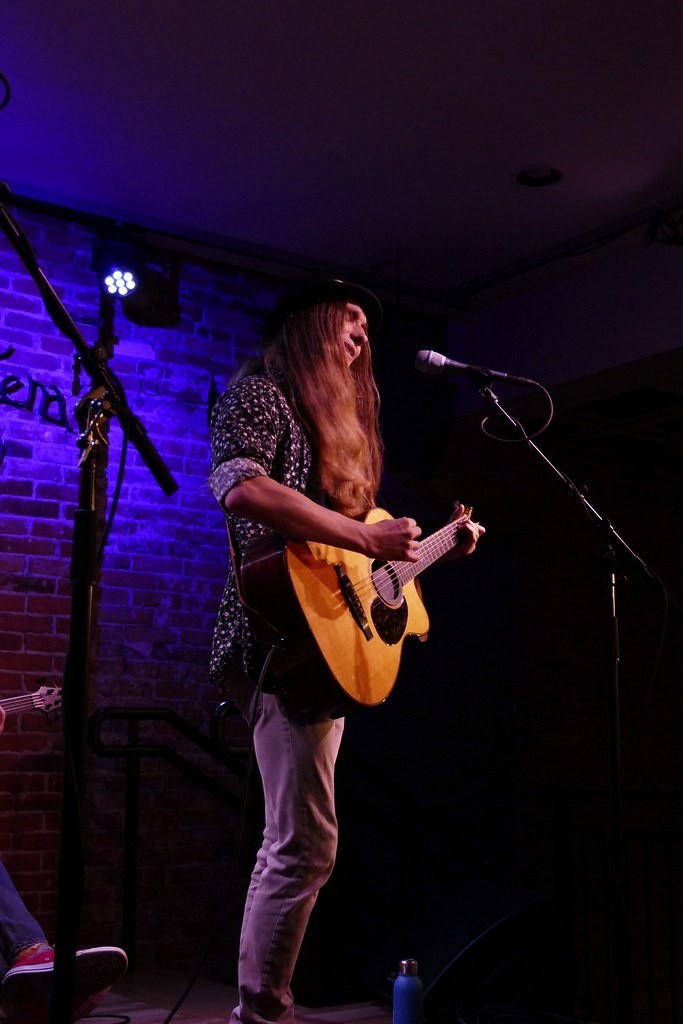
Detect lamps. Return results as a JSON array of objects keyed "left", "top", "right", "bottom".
[{"left": 95, "top": 247, "right": 139, "bottom": 297}]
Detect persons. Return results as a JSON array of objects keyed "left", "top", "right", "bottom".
[
  {"left": 209, "top": 273, "right": 486, "bottom": 1024},
  {"left": 0, "top": 861, "right": 128, "bottom": 1024}
]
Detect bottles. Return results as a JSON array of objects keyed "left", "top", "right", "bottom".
[{"left": 393, "top": 958, "right": 422, "bottom": 1024}]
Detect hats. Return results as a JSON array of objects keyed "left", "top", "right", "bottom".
[{"left": 262, "top": 272, "right": 382, "bottom": 342}]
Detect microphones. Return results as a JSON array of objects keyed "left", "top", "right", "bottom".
[{"left": 414, "top": 348, "right": 539, "bottom": 387}]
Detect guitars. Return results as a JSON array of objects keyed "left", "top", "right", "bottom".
[
  {"left": 0, "top": 676, "right": 63, "bottom": 724},
  {"left": 236, "top": 499, "right": 482, "bottom": 730}
]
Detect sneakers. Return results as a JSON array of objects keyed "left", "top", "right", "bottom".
[
  {"left": 2, "top": 943, "right": 129, "bottom": 1000},
  {"left": 0, "top": 985, "right": 112, "bottom": 1024}
]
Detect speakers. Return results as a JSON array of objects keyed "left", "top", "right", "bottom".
[{"left": 423, "top": 871, "right": 631, "bottom": 1024}]
